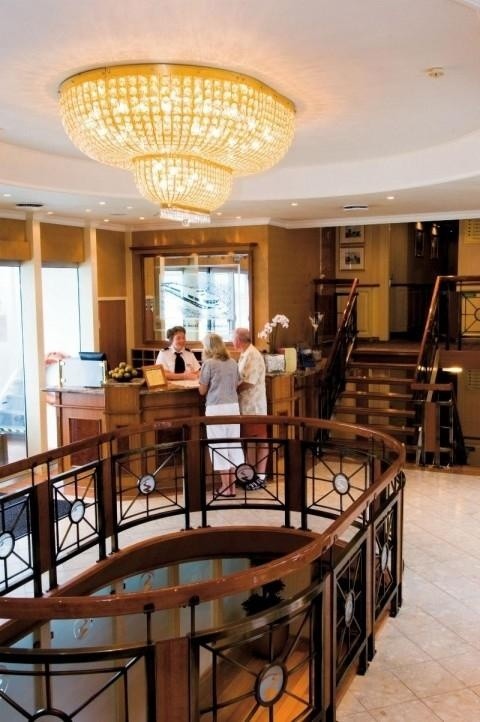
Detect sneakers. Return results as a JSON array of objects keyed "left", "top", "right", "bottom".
[{"left": 245, "top": 475, "right": 267, "bottom": 491}]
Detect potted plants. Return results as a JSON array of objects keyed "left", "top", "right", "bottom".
[{"left": 240, "top": 560, "right": 289, "bottom": 664}]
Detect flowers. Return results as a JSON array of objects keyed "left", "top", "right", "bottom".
[
  {"left": 258, "top": 314, "right": 290, "bottom": 354},
  {"left": 308, "top": 312, "right": 326, "bottom": 351}
]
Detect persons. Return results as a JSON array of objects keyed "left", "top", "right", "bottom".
[
  {"left": 156, "top": 326, "right": 201, "bottom": 381},
  {"left": 230, "top": 328, "right": 270, "bottom": 490},
  {"left": 198, "top": 332, "right": 245, "bottom": 497}
]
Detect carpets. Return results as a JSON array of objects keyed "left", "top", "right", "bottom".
[{"left": 0, "top": 493, "right": 91, "bottom": 541}]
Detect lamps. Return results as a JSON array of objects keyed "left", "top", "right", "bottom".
[{"left": 58, "top": 63, "right": 297, "bottom": 222}]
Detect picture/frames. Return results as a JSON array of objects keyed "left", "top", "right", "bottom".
[
  {"left": 414, "top": 229, "right": 425, "bottom": 257},
  {"left": 338, "top": 225, "right": 366, "bottom": 245},
  {"left": 142, "top": 364, "right": 168, "bottom": 390},
  {"left": 429, "top": 236, "right": 439, "bottom": 260},
  {"left": 339, "top": 245, "right": 365, "bottom": 271}
]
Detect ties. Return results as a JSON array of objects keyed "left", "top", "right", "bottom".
[{"left": 174, "top": 352, "right": 186, "bottom": 374}]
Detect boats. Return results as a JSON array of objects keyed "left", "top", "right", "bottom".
[{"left": 166, "top": 283, "right": 219, "bottom": 310}]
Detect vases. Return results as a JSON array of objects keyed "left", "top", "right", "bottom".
[
  {"left": 311, "top": 349, "right": 323, "bottom": 362},
  {"left": 262, "top": 353, "right": 285, "bottom": 373}
]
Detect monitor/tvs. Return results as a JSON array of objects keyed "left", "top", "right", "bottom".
[{"left": 78, "top": 351, "right": 107, "bottom": 360}]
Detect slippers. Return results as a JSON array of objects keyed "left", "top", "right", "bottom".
[{"left": 213, "top": 488, "right": 237, "bottom": 496}]
